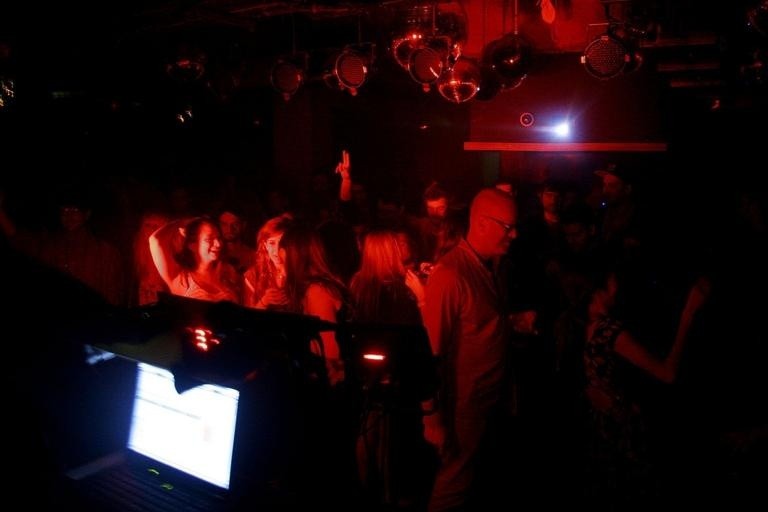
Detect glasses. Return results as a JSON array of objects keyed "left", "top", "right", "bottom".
[{"left": 487, "top": 216, "right": 514, "bottom": 231}]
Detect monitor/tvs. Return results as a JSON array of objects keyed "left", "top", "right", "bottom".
[{"left": 122, "top": 360, "right": 245, "bottom": 500}]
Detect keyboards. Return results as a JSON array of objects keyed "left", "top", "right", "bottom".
[{"left": 88, "top": 461, "right": 219, "bottom": 512}]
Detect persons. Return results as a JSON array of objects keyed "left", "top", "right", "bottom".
[
  {"left": 1, "top": 195, "right": 125, "bottom": 304},
  {"left": 128, "top": 165, "right": 766, "bottom": 512}
]
